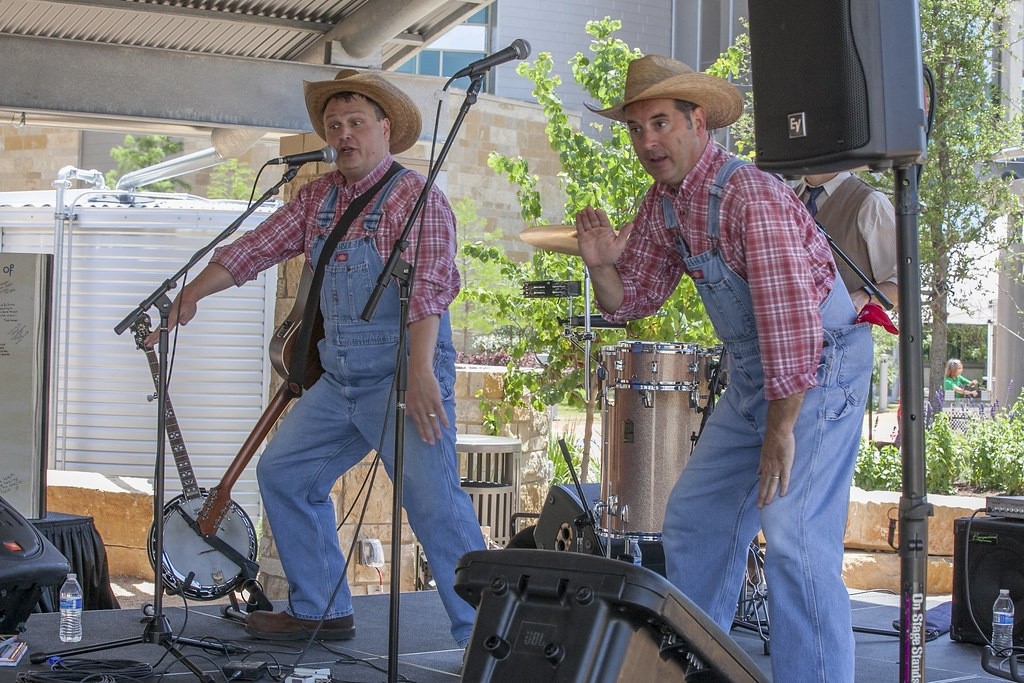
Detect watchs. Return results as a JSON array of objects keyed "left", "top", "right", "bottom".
[{"left": 859, "top": 285, "right": 875, "bottom": 303}]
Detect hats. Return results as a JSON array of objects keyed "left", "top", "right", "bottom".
[
  {"left": 303, "top": 69, "right": 422, "bottom": 156},
  {"left": 582, "top": 55, "right": 743, "bottom": 130}
]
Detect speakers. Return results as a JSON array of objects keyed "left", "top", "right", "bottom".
[
  {"left": 746, "top": 0, "right": 925, "bottom": 179},
  {"left": 949, "top": 515, "right": 1024, "bottom": 651},
  {"left": 453, "top": 548, "right": 770, "bottom": 683},
  {"left": 533, "top": 482, "right": 603, "bottom": 557},
  {"left": 0, "top": 497, "right": 71, "bottom": 635}
]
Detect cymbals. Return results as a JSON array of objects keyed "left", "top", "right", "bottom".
[{"left": 518, "top": 224, "right": 630, "bottom": 256}]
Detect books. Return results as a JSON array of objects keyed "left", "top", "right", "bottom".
[{"left": 0, "top": 635, "right": 28, "bottom": 666}]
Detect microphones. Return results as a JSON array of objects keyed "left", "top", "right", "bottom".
[
  {"left": 454, "top": 39, "right": 531, "bottom": 78},
  {"left": 267, "top": 145, "right": 337, "bottom": 166}
]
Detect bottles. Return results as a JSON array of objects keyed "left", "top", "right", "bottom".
[
  {"left": 627, "top": 539, "right": 642, "bottom": 567},
  {"left": 59, "top": 573, "right": 83, "bottom": 643},
  {"left": 991, "top": 589, "right": 1015, "bottom": 656}
]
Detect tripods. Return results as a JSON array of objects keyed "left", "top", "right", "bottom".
[{"left": 27, "top": 165, "right": 303, "bottom": 683}]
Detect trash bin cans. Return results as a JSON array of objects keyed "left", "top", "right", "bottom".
[{"left": 453, "top": 433, "right": 521, "bottom": 552}]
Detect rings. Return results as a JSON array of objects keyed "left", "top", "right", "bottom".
[
  {"left": 427, "top": 413, "right": 437, "bottom": 417},
  {"left": 771, "top": 476, "right": 781, "bottom": 478}
]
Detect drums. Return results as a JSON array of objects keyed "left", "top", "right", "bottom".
[
  {"left": 593, "top": 345, "right": 725, "bottom": 541},
  {"left": 617, "top": 340, "right": 698, "bottom": 392}
]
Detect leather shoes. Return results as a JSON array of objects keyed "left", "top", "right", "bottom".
[{"left": 244, "top": 610, "right": 356, "bottom": 641}]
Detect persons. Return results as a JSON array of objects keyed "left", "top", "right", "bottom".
[
  {"left": 576, "top": 54, "right": 875, "bottom": 683},
  {"left": 145, "top": 69, "right": 487, "bottom": 649},
  {"left": 743, "top": 170, "right": 898, "bottom": 596},
  {"left": 942, "top": 358, "right": 978, "bottom": 398}
]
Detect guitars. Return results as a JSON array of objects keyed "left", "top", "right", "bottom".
[
  {"left": 129, "top": 313, "right": 258, "bottom": 601},
  {"left": 193, "top": 257, "right": 325, "bottom": 538}
]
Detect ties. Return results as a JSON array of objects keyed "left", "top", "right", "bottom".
[{"left": 805, "top": 185, "right": 825, "bottom": 217}]
